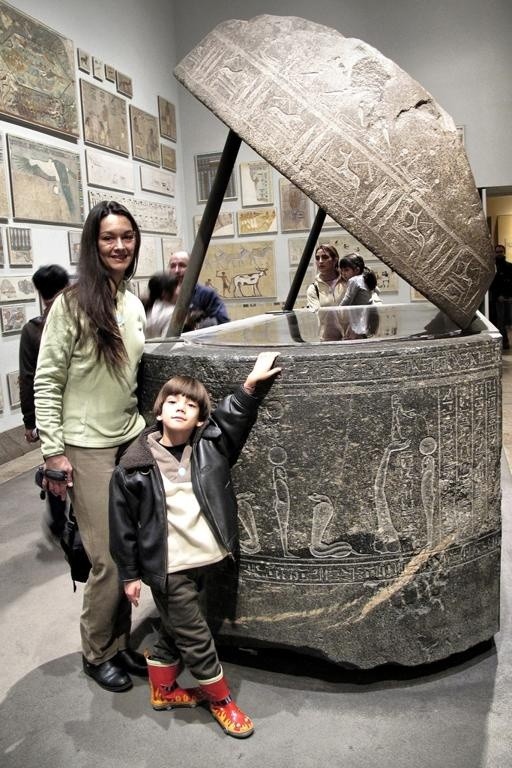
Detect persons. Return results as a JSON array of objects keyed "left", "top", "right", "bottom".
[
  {"left": 132, "top": 248, "right": 230, "bottom": 339},
  {"left": 339, "top": 253, "right": 377, "bottom": 340},
  {"left": 305, "top": 243, "right": 382, "bottom": 342},
  {"left": 34, "top": 200, "right": 148, "bottom": 695},
  {"left": 108, "top": 352, "right": 282, "bottom": 739},
  {"left": 489, "top": 245, "right": 512, "bottom": 350},
  {"left": 19, "top": 264, "right": 70, "bottom": 444}
]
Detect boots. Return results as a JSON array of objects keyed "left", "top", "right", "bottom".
[
  {"left": 145, "top": 655, "right": 206, "bottom": 711},
  {"left": 197, "top": 663, "right": 256, "bottom": 740}
]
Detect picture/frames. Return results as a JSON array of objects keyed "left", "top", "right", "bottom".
[
  {"left": 0, "top": 0, "right": 81, "bottom": 143},
  {"left": 3, "top": 305, "right": 27, "bottom": 334},
  {"left": 5, "top": 369, "right": 22, "bottom": 411},
  {"left": 407, "top": 284, "right": 428, "bottom": 303},
  {"left": 5, "top": 229, "right": 35, "bottom": 268},
  {"left": 5, "top": 131, "right": 85, "bottom": 227},
  {"left": 158, "top": 96, "right": 177, "bottom": 144},
  {"left": 87, "top": 190, "right": 177, "bottom": 236},
  {"left": 162, "top": 237, "right": 185, "bottom": 276},
  {"left": 1, "top": 275, "right": 36, "bottom": 303},
  {"left": 91, "top": 57, "right": 103, "bottom": 82},
  {"left": 115, "top": 70, "right": 132, "bottom": 96},
  {"left": 104, "top": 64, "right": 115, "bottom": 82},
  {"left": 137, "top": 166, "right": 175, "bottom": 198},
  {"left": 79, "top": 80, "right": 132, "bottom": 158},
  {"left": 77, "top": 48, "right": 91, "bottom": 73},
  {"left": 160, "top": 143, "right": 176, "bottom": 172},
  {"left": 84, "top": 148, "right": 136, "bottom": 194},
  {"left": 192, "top": 150, "right": 402, "bottom": 307},
  {"left": 129, "top": 105, "right": 160, "bottom": 167},
  {"left": 133, "top": 237, "right": 161, "bottom": 278},
  {"left": 69, "top": 230, "right": 85, "bottom": 264}
]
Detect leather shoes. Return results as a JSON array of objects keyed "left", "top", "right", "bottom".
[
  {"left": 81, "top": 653, "right": 133, "bottom": 692},
  {"left": 110, "top": 649, "right": 149, "bottom": 677}
]
